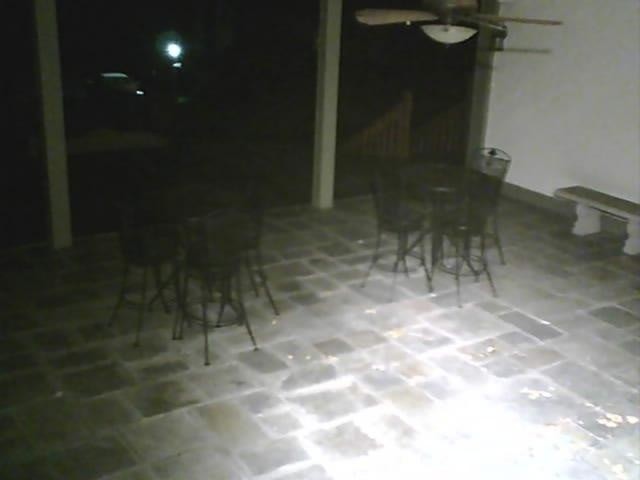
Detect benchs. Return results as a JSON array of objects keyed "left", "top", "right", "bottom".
[{"left": 555, "top": 185, "right": 640, "bottom": 257}]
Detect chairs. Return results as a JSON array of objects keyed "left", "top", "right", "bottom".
[
  {"left": 106, "top": 182, "right": 279, "bottom": 370},
  {"left": 366, "top": 146, "right": 512, "bottom": 293}
]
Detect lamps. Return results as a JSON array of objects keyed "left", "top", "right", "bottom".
[{"left": 418, "top": 19, "right": 479, "bottom": 44}]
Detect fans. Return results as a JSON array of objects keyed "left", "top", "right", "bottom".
[{"left": 353, "top": 2, "right": 564, "bottom": 30}]
